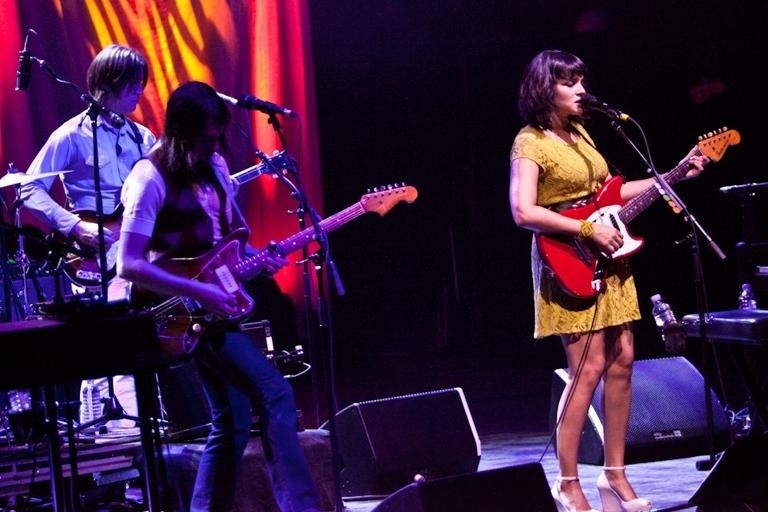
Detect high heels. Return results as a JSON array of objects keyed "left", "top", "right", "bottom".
[
  {"left": 549, "top": 476, "right": 594, "bottom": 512},
  {"left": 594, "top": 464, "right": 652, "bottom": 512}
]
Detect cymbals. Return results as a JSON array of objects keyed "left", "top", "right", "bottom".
[{"left": 0, "top": 169, "right": 77, "bottom": 189}]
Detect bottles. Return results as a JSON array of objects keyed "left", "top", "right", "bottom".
[
  {"left": 737, "top": 283, "right": 758, "bottom": 314},
  {"left": 651, "top": 294, "right": 686, "bottom": 355}
]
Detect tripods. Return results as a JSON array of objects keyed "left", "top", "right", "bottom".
[{"left": 42, "top": 59, "right": 175, "bottom": 437}]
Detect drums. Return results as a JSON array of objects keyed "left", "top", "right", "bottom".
[{"left": 0, "top": 319, "right": 74, "bottom": 393}]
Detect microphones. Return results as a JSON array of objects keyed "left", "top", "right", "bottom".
[
  {"left": 233, "top": 90, "right": 297, "bottom": 118},
  {"left": 580, "top": 92, "right": 628, "bottom": 122},
  {"left": 15, "top": 29, "right": 39, "bottom": 91}
]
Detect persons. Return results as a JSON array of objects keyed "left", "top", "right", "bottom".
[
  {"left": 507, "top": 52, "right": 709, "bottom": 508},
  {"left": 15, "top": 46, "right": 157, "bottom": 299},
  {"left": 116, "top": 82, "right": 325, "bottom": 509}
]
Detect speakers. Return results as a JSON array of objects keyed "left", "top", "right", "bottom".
[
  {"left": 319, "top": 386, "right": 481, "bottom": 495},
  {"left": 371, "top": 462, "right": 558, "bottom": 512},
  {"left": 552, "top": 355, "right": 735, "bottom": 465}
]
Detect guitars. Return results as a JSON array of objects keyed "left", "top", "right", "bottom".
[
  {"left": 53, "top": 149, "right": 299, "bottom": 289},
  {"left": 535, "top": 127, "right": 740, "bottom": 300},
  {"left": 127, "top": 182, "right": 418, "bottom": 370}
]
